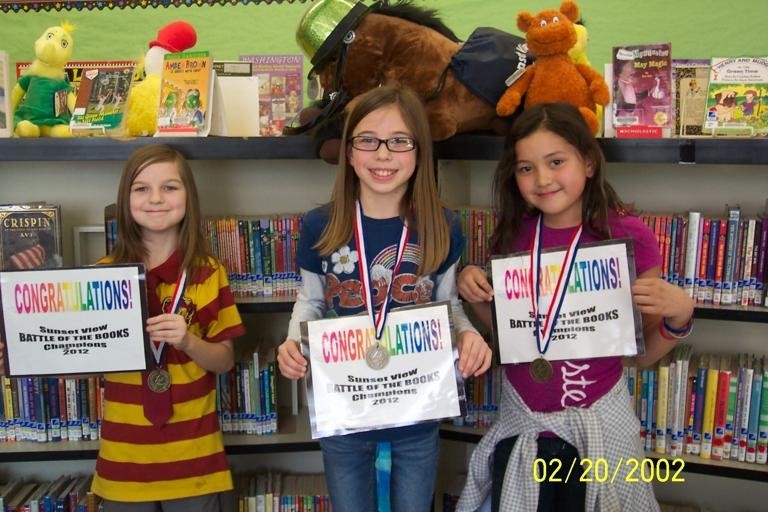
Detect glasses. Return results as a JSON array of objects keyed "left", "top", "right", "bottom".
[{"left": 347, "top": 135, "right": 416, "bottom": 153}]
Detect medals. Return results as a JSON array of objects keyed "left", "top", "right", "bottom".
[
  {"left": 364, "top": 343, "right": 390, "bottom": 370},
  {"left": 147, "top": 368, "right": 172, "bottom": 393},
  {"left": 530, "top": 356, "right": 555, "bottom": 383}
]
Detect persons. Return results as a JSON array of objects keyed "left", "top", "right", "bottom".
[
  {"left": 456, "top": 100, "right": 693, "bottom": 512},
  {"left": 278, "top": 87, "right": 492, "bottom": 512},
  {"left": 0, "top": 149, "right": 245, "bottom": 511}
]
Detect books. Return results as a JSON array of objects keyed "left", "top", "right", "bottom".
[
  {"left": 609, "top": 43, "right": 768, "bottom": 139},
  {"left": 1, "top": 199, "right": 118, "bottom": 272},
  {"left": 0, "top": 375, "right": 102, "bottom": 512},
  {"left": 156, "top": 50, "right": 214, "bottom": 138},
  {"left": 69, "top": 65, "right": 135, "bottom": 130},
  {"left": 624, "top": 203, "right": 768, "bottom": 468},
  {"left": 432, "top": 208, "right": 502, "bottom": 511},
  {"left": 204, "top": 212, "right": 330, "bottom": 512}
]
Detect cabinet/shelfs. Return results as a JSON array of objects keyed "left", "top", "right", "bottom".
[{"left": 1, "top": 138, "right": 768, "bottom": 512}]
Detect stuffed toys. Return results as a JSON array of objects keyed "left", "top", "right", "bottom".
[
  {"left": 283, "top": 0, "right": 611, "bottom": 164},
  {"left": 284, "top": 0, "right": 535, "bottom": 163},
  {"left": 10, "top": 23, "right": 77, "bottom": 138},
  {"left": 119, "top": 18, "right": 197, "bottom": 138},
  {"left": 496, "top": 0, "right": 610, "bottom": 138}
]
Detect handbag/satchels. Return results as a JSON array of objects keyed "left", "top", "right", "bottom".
[{"left": 451, "top": 26, "right": 539, "bottom": 121}]
